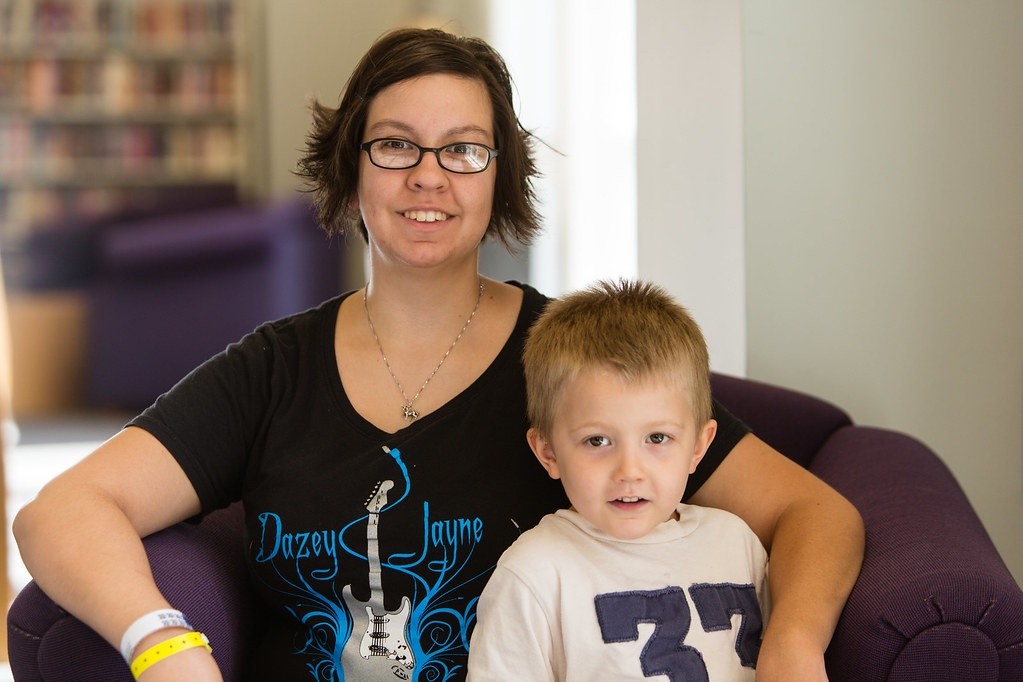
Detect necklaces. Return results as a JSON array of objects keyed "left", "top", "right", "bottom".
[{"left": 364, "top": 279, "right": 485, "bottom": 421}]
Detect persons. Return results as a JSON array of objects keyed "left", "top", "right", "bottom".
[
  {"left": 464, "top": 281, "right": 772, "bottom": 682},
  {"left": 14, "top": 27, "right": 866, "bottom": 682}
]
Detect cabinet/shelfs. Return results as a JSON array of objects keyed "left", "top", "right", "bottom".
[{"left": 0, "top": 0, "right": 265, "bottom": 229}]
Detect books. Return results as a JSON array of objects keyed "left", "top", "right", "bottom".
[{"left": 0, "top": 1, "right": 241, "bottom": 240}]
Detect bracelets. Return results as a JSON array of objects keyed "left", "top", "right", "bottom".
[
  {"left": 120, "top": 609, "right": 194, "bottom": 663},
  {"left": 129, "top": 632, "right": 213, "bottom": 680}
]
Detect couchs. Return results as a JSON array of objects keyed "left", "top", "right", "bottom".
[{"left": 6, "top": 183, "right": 1023, "bottom": 682}]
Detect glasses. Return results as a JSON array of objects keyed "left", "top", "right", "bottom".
[{"left": 360, "top": 137, "right": 500, "bottom": 175}]
